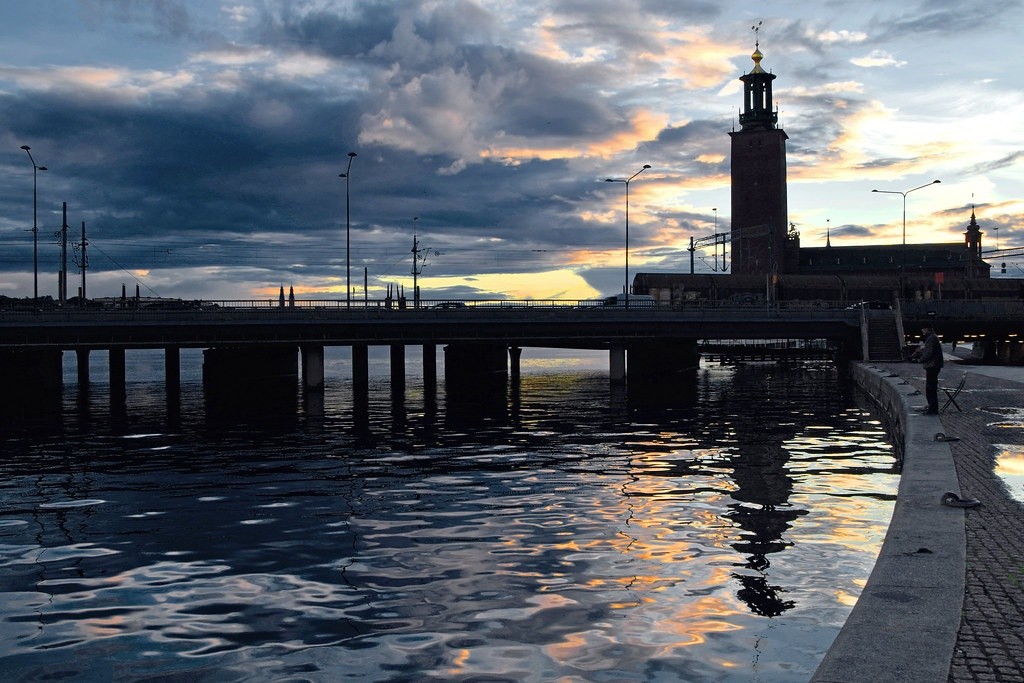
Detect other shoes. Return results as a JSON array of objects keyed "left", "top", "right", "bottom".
[{"left": 921, "top": 409, "right": 938, "bottom": 415}]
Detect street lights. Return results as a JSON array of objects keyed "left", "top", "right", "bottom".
[
  {"left": 19, "top": 144, "right": 49, "bottom": 298},
  {"left": 872, "top": 179, "right": 941, "bottom": 243},
  {"left": 339, "top": 151, "right": 356, "bottom": 306},
  {"left": 605, "top": 164, "right": 652, "bottom": 306}
]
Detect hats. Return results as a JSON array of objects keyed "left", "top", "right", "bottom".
[{"left": 920, "top": 321, "right": 932, "bottom": 329}]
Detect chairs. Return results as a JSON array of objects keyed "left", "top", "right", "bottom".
[{"left": 940, "top": 371, "right": 968, "bottom": 412}]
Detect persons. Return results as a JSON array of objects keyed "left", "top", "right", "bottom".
[
  {"left": 952, "top": 337, "right": 957, "bottom": 351},
  {"left": 917, "top": 321, "right": 943, "bottom": 416}
]
